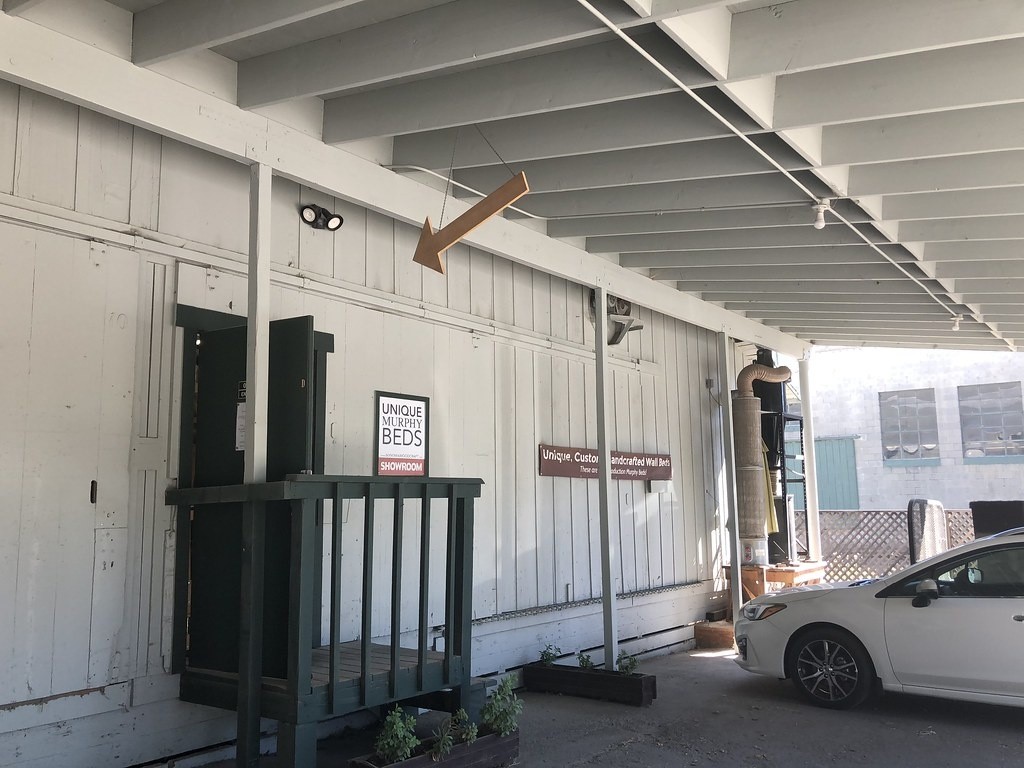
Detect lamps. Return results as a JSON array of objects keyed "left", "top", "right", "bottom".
[{"left": 301, "top": 204, "right": 344, "bottom": 231}]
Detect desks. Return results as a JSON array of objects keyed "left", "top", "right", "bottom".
[{"left": 722, "top": 561, "right": 825, "bottom": 621}]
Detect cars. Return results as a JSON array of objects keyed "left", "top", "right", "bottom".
[{"left": 733, "top": 527, "right": 1024, "bottom": 713}]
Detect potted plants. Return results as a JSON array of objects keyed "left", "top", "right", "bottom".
[
  {"left": 523, "top": 644, "right": 657, "bottom": 706},
  {"left": 348, "top": 673, "right": 524, "bottom": 768}
]
